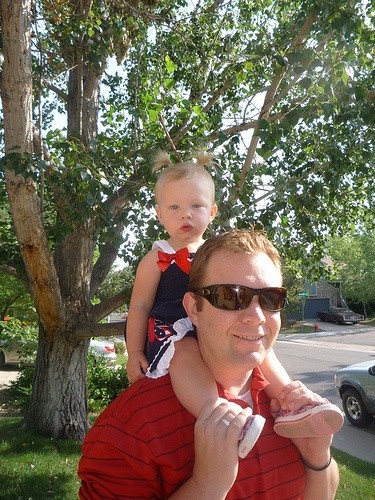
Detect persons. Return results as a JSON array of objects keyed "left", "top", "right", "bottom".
[
  {"left": 76, "top": 229, "right": 339, "bottom": 500},
  {"left": 124, "top": 149, "right": 345, "bottom": 459}
]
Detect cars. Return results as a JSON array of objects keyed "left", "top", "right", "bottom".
[
  {"left": 0, "top": 338, "right": 119, "bottom": 370},
  {"left": 317, "top": 307, "right": 364, "bottom": 324},
  {"left": 335, "top": 360, "right": 375, "bottom": 426}
]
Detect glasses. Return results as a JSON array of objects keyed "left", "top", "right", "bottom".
[{"left": 188, "top": 283, "right": 290, "bottom": 313}]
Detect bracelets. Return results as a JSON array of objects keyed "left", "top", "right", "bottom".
[{"left": 301, "top": 455, "right": 332, "bottom": 471}]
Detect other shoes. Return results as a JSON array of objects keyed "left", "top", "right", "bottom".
[{"left": 238, "top": 400, "right": 344, "bottom": 459}]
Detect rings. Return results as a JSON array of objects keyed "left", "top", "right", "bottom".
[{"left": 221, "top": 418, "right": 230, "bottom": 426}]
